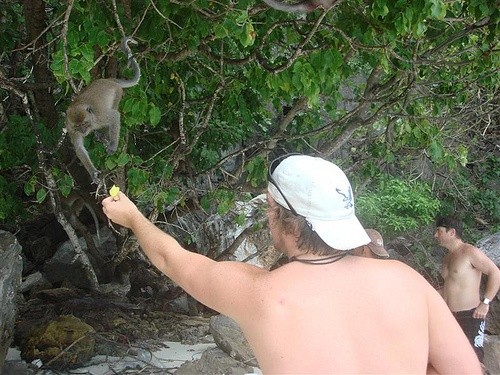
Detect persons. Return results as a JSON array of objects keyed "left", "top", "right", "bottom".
[
  {"left": 433, "top": 216, "right": 500, "bottom": 367},
  {"left": 101, "top": 154, "right": 484, "bottom": 375},
  {"left": 353, "top": 229, "right": 389, "bottom": 258}
]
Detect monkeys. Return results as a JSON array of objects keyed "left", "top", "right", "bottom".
[{"left": 66, "top": 37, "right": 141, "bottom": 184}]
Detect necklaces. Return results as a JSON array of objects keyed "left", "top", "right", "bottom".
[{"left": 291, "top": 252, "right": 348, "bottom": 266}]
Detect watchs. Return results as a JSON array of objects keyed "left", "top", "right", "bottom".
[{"left": 481, "top": 297, "right": 493, "bottom": 305}]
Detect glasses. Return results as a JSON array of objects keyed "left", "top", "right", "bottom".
[{"left": 267, "top": 152, "right": 302, "bottom": 216}]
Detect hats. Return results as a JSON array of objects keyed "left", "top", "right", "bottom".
[
  {"left": 268, "top": 155, "right": 371, "bottom": 250},
  {"left": 364, "top": 229, "right": 389, "bottom": 258}
]
